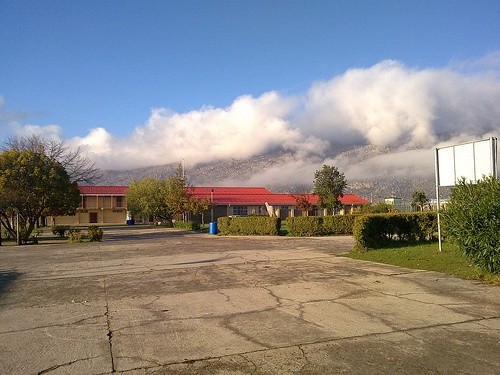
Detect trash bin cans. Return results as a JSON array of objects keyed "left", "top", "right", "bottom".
[{"left": 209, "top": 221, "right": 218, "bottom": 235}]
